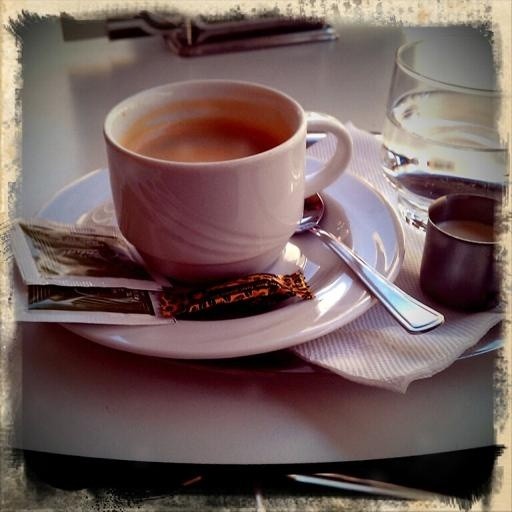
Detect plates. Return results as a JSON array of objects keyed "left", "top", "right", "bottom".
[{"left": 27, "top": 154, "right": 405, "bottom": 360}]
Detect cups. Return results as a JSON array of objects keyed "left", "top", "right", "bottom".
[
  {"left": 421, "top": 193, "right": 502, "bottom": 312},
  {"left": 101, "top": 77, "right": 354, "bottom": 281},
  {"left": 384, "top": 35, "right": 511, "bottom": 212}
]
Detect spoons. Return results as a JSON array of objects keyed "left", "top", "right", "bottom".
[{"left": 293, "top": 189, "right": 448, "bottom": 335}]
